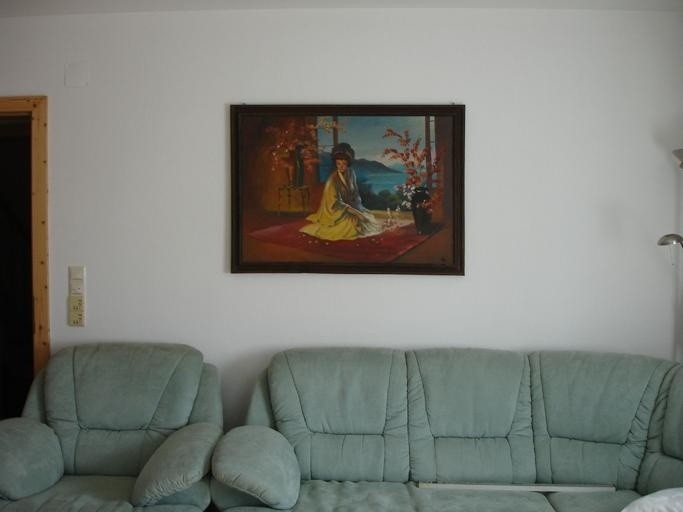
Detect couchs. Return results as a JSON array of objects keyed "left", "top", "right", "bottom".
[{"left": 211, "top": 344, "right": 683, "bottom": 512}]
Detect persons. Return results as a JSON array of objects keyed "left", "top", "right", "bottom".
[{"left": 299, "top": 143, "right": 385, "bottom": 241}]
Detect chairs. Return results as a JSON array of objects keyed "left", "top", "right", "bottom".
[{"left": 0, "top": 341, "right": 224, "bottom": 511}]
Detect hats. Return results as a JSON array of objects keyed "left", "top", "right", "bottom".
[{"left": 331, "top": 143, "right": 355, "bottom": 160}]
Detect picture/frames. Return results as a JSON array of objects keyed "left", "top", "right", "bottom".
[{"left": 230, "top": 104, "right": 465, "bottom": 275}]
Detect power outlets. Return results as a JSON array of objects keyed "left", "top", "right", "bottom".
[{"left": 68, "top": 294, "right": 85, "bottom": 327}]
